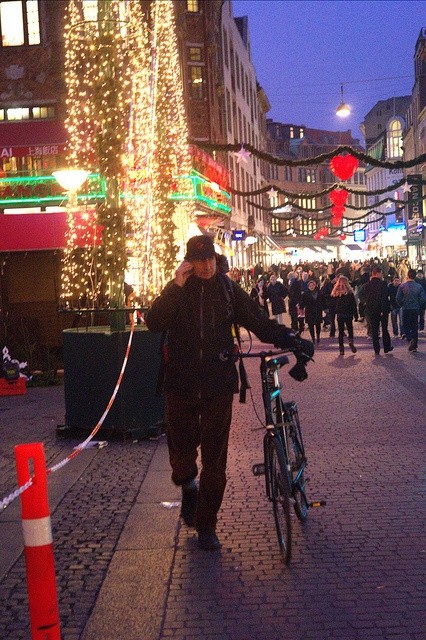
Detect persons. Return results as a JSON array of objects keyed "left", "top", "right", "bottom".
[
  {"left": 356, "top": 255, "right": 426, "bottom": 354},
  {"left": 146, "top": 237, "right": 290, "bottom": 549},
  {"left": 294, "top": 254, "right": 356, "bottom": 354},
  {"left": 227, "top": 261, "right": 294, "bottom": 330}
]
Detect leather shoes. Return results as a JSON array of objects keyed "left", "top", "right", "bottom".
[
  {"left": 180, "top": 486, "right": 200, "bottom": 528},
  {"left": 384, "top": 346, "right": 394, "bottom": 353},
  {"left": 375, "top": 350, "right": 380, "bottom": 354},
  {"left": 197, "top": 524, "right": 221, "bottom": 551}
]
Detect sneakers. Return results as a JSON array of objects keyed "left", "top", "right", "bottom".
[
  {"left": 408, "top": 346, "right": 417, "bottom": 352},
  {"left": 323, "top": 324, "right": 328, "bottom": 330},
  {"left": 418, "top": 331, "right": 425, "bottom": 336},
  {"left": 401, "top": 333, "right": 407, "bottom": 340},
  {"left": 367, "top": 336, "right": 372, "bottom": 340}
]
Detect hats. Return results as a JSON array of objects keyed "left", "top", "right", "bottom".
[
  {"left": 307, "top": 277, "right": 317, "bottom": 288},
  {"left": 186, "top": 234, "right": 215, "bottom": 258}
]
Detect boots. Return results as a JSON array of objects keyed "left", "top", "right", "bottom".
[
  {"left": 349, "top": 342, "right": 357, "bottom": 353},
  {"left": 339, "top": 346, "right": 345, "bottom": 355},
  {"left": 312, "top": 336, "right": 315, "bottom": 344},
  {"left": 317, "top": 334, "right": 320, "bottom": 342}
]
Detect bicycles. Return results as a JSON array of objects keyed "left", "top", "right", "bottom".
[{"left": 218, "top": 339, "right": 329, "bottom": 565}]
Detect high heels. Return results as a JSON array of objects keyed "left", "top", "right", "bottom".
[{"left": 298, "top": 328, "right": 305, "bottom": 334}]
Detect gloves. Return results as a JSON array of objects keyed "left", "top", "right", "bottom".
[
  {"left": 289, "top": 353, "right": 308, "bottom": 382},
  {"left": 289, "top": 333, "right": 314, "bottom": 360}
]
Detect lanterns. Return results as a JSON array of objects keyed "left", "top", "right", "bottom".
[
  {"left": 313, "top": 231, "right": 320, "bottom": 239},
  {"left": 332, "top": 204, "right": 346, "bottom": 216},
  {"left": 332, "top": 217, "right": 340, "bottom": 226},
  {"left": 329, "top": 154, "right": 359, "bottom": 180},
  {"left": 329, "top": 188, "right": 349, "bottom": 207},
  {"left": 339, "top": 234, "right": 346, "bottom": 240},
  {"left": 320, "top": 226, "right": 329, "bottom": 237}
]
